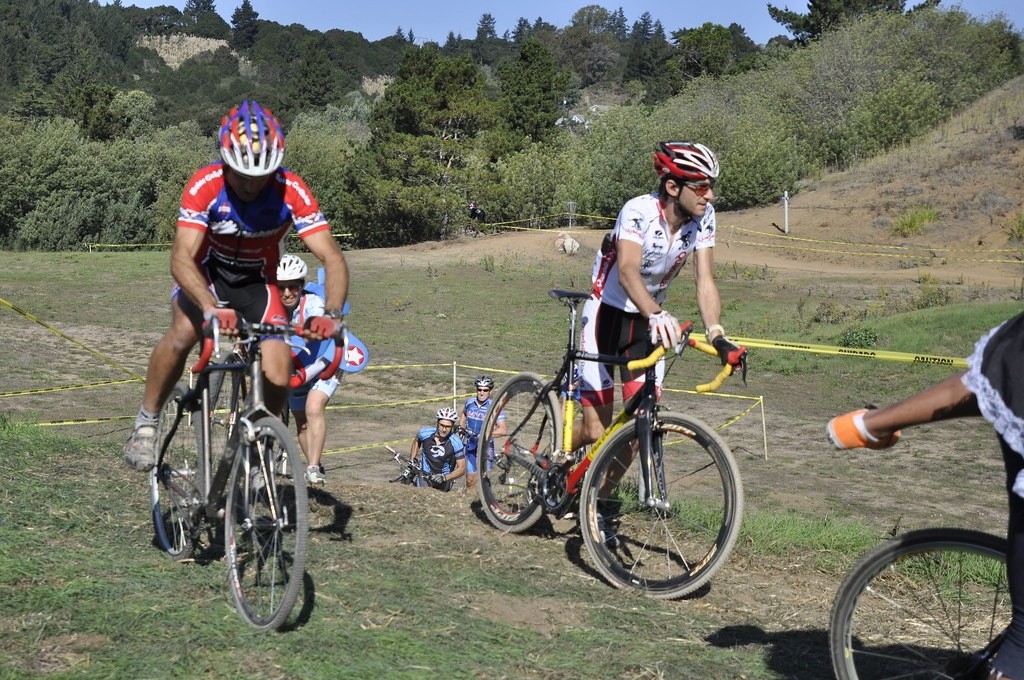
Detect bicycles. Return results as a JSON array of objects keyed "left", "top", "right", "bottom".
[
  {"left": 384, "top": 444, "right": 434, "bottom": 488},
  {"left": 148, "top": 313, "right": 347, "bottom": 634},
  {"left": 827, "top": 526, "right": 1017, "bottom": 680},
  {"left": 476, "top": 287, "right": 749, "bottom": 604}
]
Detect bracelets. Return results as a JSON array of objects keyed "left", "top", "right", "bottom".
[
  {"left": 324, "top": 308, "right": 343, "bottom": 321},
  {"left": 705, "top": 324, "right": 724, "bottom": 340}
]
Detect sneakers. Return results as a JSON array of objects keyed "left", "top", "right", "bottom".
[
  {"left": 123, "top": 424, "right": 156, "bottom": 472},
  {"left": 592, "top": 504, "right": 620, "bottom": 548},
  {"left": 826, "top": 408, "right": 900, "bottom": 450},
  {"left": 304, "top": 465, "right": 327, "bottom": 484}
]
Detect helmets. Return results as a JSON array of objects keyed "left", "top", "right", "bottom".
[
  {"left": 217, "top": 98, "right": 286, "bottom": 179},
  {"left": 654, "top": 141, "right": 720, "bottom": 225},
  {"left": 474, "top": 375, "right": 495, "bottom": 389},
  {"left": 436, "top": 407, "right": 458, "bottom": 422},
  {"left": 276, "top": 254, "right": 308, "bottom": 280}
]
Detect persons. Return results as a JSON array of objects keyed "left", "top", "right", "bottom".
[
  {"left": 826, "top": 310, "right": 1024, "bottom": 680},
  {"left": 124, "top": 102, "right": 347, "bottom": 541},
  {"left": 276, "top": 254, "right": 344, "bottom": 483},
  {"left": 469, "top": 207, "right": 485, "bottom": 222},
  {"left": 460, "top": 375, "right": 506, "bottom": 490},
  {"left": 409, "top": 407, "right": 466, "bottom": 492},
  {"left": 527, "top": 138, "right": 741, "bottom": 546}
]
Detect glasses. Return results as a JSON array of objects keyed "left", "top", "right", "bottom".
[
  {"left": 685, "top": 181, "right": 715, "bottom": 198},
  {"left": 278, "top": 285, "right": 299, "bottom": 294},
  {"left": 438, "top": 422, "right": 453, "bottom": 428},
  {"left": 478, "top": 389, "right": 490, "bottom": 392}
]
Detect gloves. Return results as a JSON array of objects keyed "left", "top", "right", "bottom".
[
  {"left": 647, "top": 309, "right": 681, "bottom": 350},
  {"left": 431, "top": 474, "right": 446, "bottom": 484},
  {"left": 711, "top": 335, "right": 748, "bottom": 376}
]
